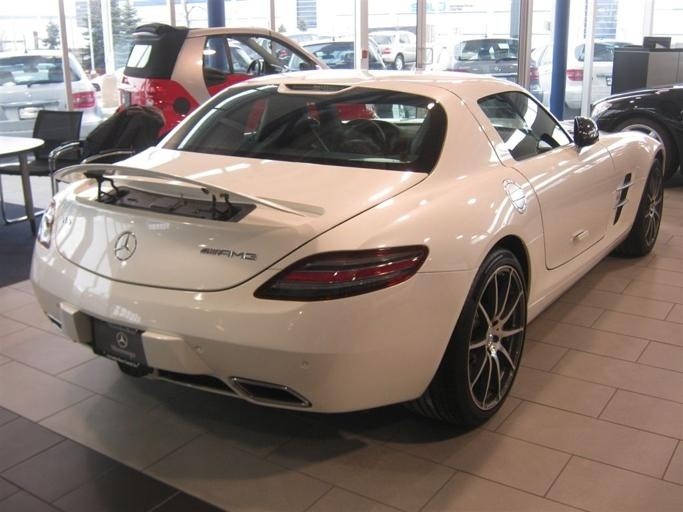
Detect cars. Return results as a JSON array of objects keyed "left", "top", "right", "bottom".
[
  {"left": 1, "top": 49, "right": 105, "bottom": 164},
  {"left": 89, "top": 62, "right": 122, "bottom": 93},
  {"left": 533, "top": 38, "right": 636, "bottom": 111},
  {"left": 249, "top": 31, "right": 383, "bottom": 72},
  {"left": 118, "top": 21, "right": 373, "bottom": 145},
  {"left": 438, "top": 35, "right": 543, "bottom": 103},
  {"left": 590, "top": 83, "right": 683, "bottom": 181},
  {"left": 368, "top": 28, "right": 433, "bottom": 71},
  {"left": 287, "top": 40, "right": 385, "bottom": 71}
]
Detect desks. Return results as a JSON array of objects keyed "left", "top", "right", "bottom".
[{"left": 0, "top": 135, "right": 45, "bottom": 236}]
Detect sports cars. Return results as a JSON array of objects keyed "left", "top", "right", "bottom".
[{"left": 27, "top": 68, "right": 667, "bottom": 429}]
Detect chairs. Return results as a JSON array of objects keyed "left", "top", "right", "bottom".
[
  {"left": 341, "top": 117, "right": 388, "bottom": 154},
  {"left": 49, "top": 104, "right": 165, "bottom": 196},
  {"left": 47, "top": 66, "right": 78, "bottom": 80},
  {"left": 0, "top": 110, "right": 84, "bottom": 224}
]
